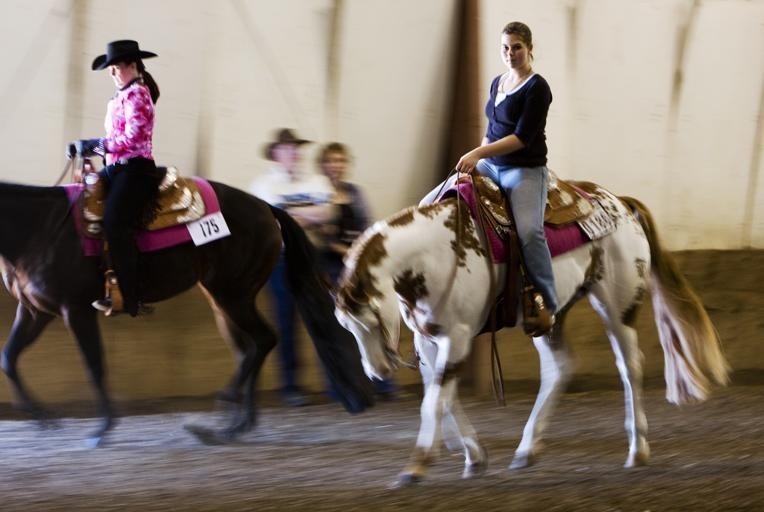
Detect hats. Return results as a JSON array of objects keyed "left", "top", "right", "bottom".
[
  {"left": 264, "top": 127, "right": 312, "bottom": 160},
  {"left": 91, "top": 40, "right": 157, "bottom": 71}
]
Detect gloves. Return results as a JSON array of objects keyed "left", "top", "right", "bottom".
[{"left": 68, "top": 137, "right": 106, "bottom": 159}]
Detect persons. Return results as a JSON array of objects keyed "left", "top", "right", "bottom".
[
  {"left": 453, "top": 18, "right": 561, "bottom": 334},
  {"left": 247, "top": 126, "right": 344, "bottom": 407},
  {"left": 64, "top": 38, "right": 162, "bottom": 320},
  {"left": 315, "top": 139, "right": 399, "bottom": 400}
]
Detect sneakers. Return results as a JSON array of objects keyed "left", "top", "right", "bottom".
[
  {"left": 522, "top": 310, "right": 557, "bottom": 328},
  {"left": 92, "top": 298, "right": 143, "bottom": 314},
  {"left": 281, "top": 390, "right": 310, "bottom": 406}
]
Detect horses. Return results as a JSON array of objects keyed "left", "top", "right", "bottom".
[
  {"left": 0, "top": 175, "right": 373, "bottom": 444},
  {"left": 328, "top": 176, "right": 735, "bottom": 493}
]
